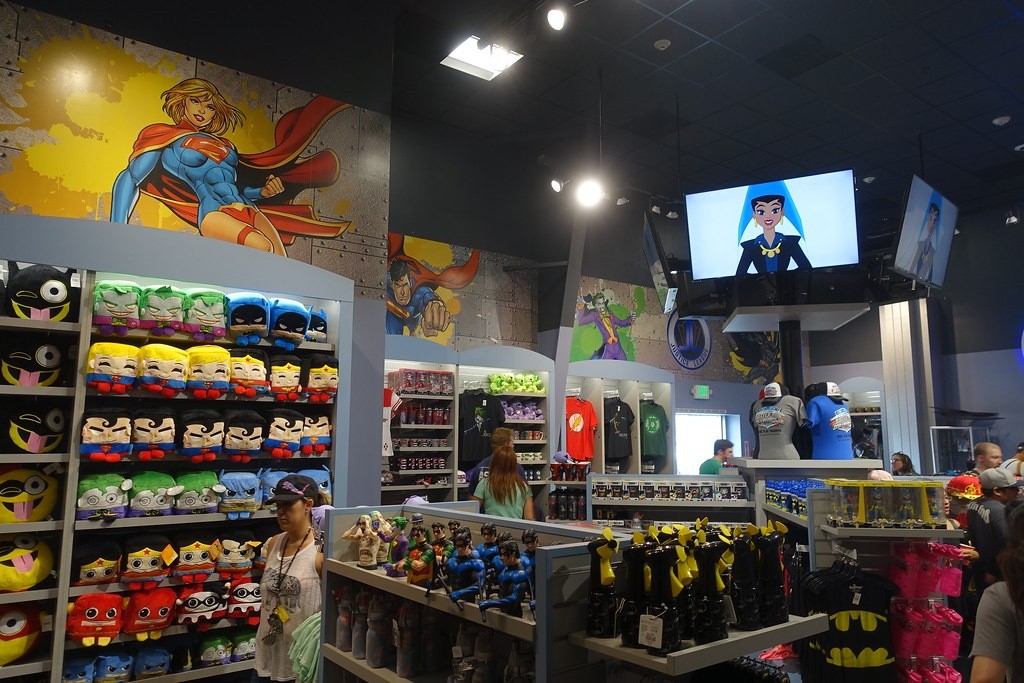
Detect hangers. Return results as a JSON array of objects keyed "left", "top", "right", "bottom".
[
  {"left": 614, "top": 392, "right": 622, "bottom": 403},
  {"left": 796, "top": 543, "right": 873, "bottom": 595},
  {"left": 646, "top": 393, "right": 656, "bottom": 406},
  {"left": 574, "top": 388, "right": 584, "bottom": 403}
]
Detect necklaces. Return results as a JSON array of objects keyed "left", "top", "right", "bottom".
[{"left": 261, "top": 527, "right": 313, "bottom": 646}]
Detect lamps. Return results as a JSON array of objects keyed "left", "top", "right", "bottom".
[
  {"left": 1003, "top": 209, "right": 1018, "bottom": 225},
  {"left": 478, "top": 0, "right": 567, "bottom": 52},
  {"left": 690, "top": 384, "right": 713, "bottom": 400},
  {"left": 551, "top": 67, "right": 685, "bottom": 218}
]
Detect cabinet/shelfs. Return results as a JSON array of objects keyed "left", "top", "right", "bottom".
[
  {"left": 379, "top": 363, "right": 549, "bottom": 502},
  {"left": 0, "top": 268, "right": 335, "bottom": 682},
  {"left": 586, "top": 459, "right": 965, "bottom": 574},
  {"left": 318, "top": 500, "right": 830, "bottom": 683},
  {"left": 929, "top": 425, "right": 991, "bottom": 474}
]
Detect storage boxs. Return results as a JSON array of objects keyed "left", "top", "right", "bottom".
[{"left": 388, "top": 368, "right": 453, "bottom": 396}]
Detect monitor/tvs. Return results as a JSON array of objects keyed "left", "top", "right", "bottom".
[{"left": 641, "top": 167, "right": 958, "bottom": 314}]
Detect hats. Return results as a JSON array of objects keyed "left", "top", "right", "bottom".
[
  {"left": 265, "top": 474, "right": 319, "bottom": 505},
  {"left": 945, "top": 473, "right": 985, "bottom": 501},
  {"left": 1005, "top": 499, "right": 1024, "bottom": 537},
  {"left": 1018, "top": 442, "right": 1024, "bottom": 449},
  {"left": 978, "top": 467, "right": 1024, "bottom": 489},
  {"left": 814, "top": 381, "right": 849, "bottom": 402},
  {"left": 759, "top": 388, "right": 764, "bottom": 400},
  {"left": 761, "top": 381, "right": 790, "bottom": 404},
  {"left": 553, "top": 451, "right": 573, "bottom": 463},
  {"left": 805, "top": 383, "right": 816, "bottom": 400}
]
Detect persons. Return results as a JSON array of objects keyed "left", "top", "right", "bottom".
[
  {"left": 866, "top": 486, "right": 940, "bottom": 522},
  {"left": 890, "top": 451, "right": 920, "bottom": 476},
  {"left": 469, "top": 427, "right": 536, "bottom": 521},
  {"left": 342, "top": 511, "right": 543, "bottom": 623},
  {"left": 943, "top": 442, "right": 1024, "bottom": 683},
  {"left": 750, "top": 381, "right": 853, "bottom": 461},
  {"left": 701, "top": 439, "right": 734, "bottom": 474},
  {"left": 251, "top": 475, "right": 323, "bottom": 683}
]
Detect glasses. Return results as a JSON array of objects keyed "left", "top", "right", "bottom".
[
  {"left": 945, "top": 496, "right": 953, "bottom": 501},
  {"left": 890, "top": 459, "right": 902, "bottom": 463}
]
